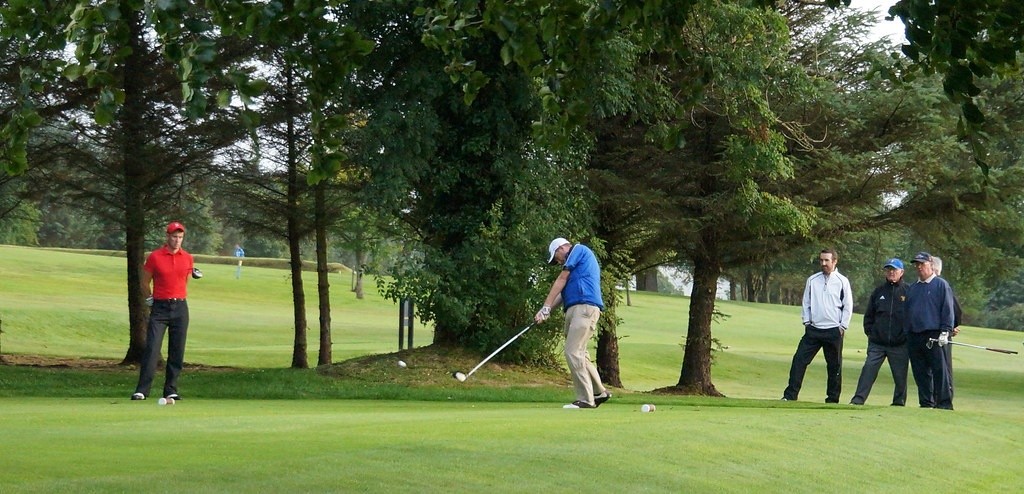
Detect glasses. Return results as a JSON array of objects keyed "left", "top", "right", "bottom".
[
  {"left": 884, "top": 266, "right": 896, "bottom": 271},
  {"left": 913, "top": 262, "right": 925, "bottom": 267}
]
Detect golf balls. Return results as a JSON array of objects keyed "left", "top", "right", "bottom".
[
  {"left": 641, "top": 403, "right": 656, "bottom": 412},
  {"left": 158, "top": 398, "right": 175, "bottom": 405}
]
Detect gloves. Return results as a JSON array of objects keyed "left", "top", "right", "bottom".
[
  {"left": 146, "top": 297, "right": 154, "bottom": 307},
  {"left": 938, "top": 332, "right": 948, "bottom": 347},
  {"left": 540, "top": 305, "right": 552, "bottom": 321}
]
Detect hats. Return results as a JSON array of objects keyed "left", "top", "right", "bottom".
[
  {"left": 548, "top": 237, "right": 570, "bottom": 267},
  {"left": 910, "top": 252, "right": 932, "bottom": 263},
  {"left": 168, "top": 223, "right": 185, "bottom": 234},
  {"left": 883, "top": 258, "right": 904, "bottom": 270}
]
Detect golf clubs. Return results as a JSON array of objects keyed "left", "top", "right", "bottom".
[
  {"left": 926, "top": 337, "right": 1019, "bottom": 355},
  {"left": 452, "top": 321, "right": 536, "bottom": 382}
]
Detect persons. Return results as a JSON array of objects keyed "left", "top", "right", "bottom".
[
  {"left": 234, "top": 244, "right": 244, "bottom": 257},
  {"left": 903, "top": 253, "right": 954, "bottom": 409},
  {"left": 534, "top": 238, "right": 612, "bottom": 408},
  {"left": 130, "top": 222, "right": 193, "bottom": 400},
  {"left": 931, "top": 256, "right": 962, "bottom": 401},
  {"left": 849, "top": 259, "right": 910, "bottom": 406},
  {"left": 780, "top": 249, "right": 854, "bottom": 403}
]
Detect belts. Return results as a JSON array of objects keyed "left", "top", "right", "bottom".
[{"left": 162, "top": 298, "right": 187, "bottom": 303}]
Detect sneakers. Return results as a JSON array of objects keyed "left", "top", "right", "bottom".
[
  {"left": 563, "top": 398, "right": 595, "bottom": 408},
  {"left": 595, "top": 390, "right": 612, "bottom": 408},
  {"left": 132, "top": 392, "right": 145, "bottom": 400},
  {"left": 166, "top": 393, "right": 181, "bottom": 400}
]
